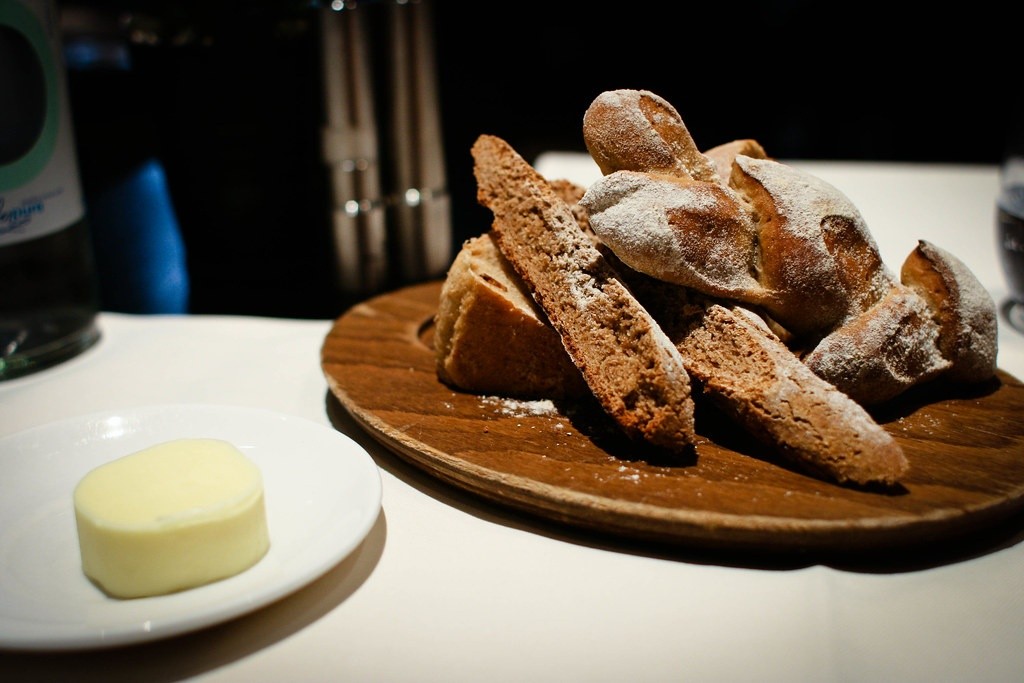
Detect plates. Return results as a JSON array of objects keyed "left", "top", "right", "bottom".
[
  {"left": 2, "top": 400, "right": 382, "bottom": 647},
  {"left": 321, "top": 282, "right": 1023, "bottom": 538}
]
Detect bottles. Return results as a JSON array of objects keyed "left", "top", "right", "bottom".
[{"left": 1, "top": 1, "right": 102, "bottom": 383}]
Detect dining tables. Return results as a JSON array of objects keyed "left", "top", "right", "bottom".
[{"left": 0, "top": 312, "right": 1024, "bottom": 683}]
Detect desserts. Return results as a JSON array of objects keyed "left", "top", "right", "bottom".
[{"left": 74, "top": 438, "right": 269, "bottom": 598}]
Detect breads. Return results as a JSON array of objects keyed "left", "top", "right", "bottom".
[{"left": 433, "top": 87, "right": 998, "bottom": 486}]
